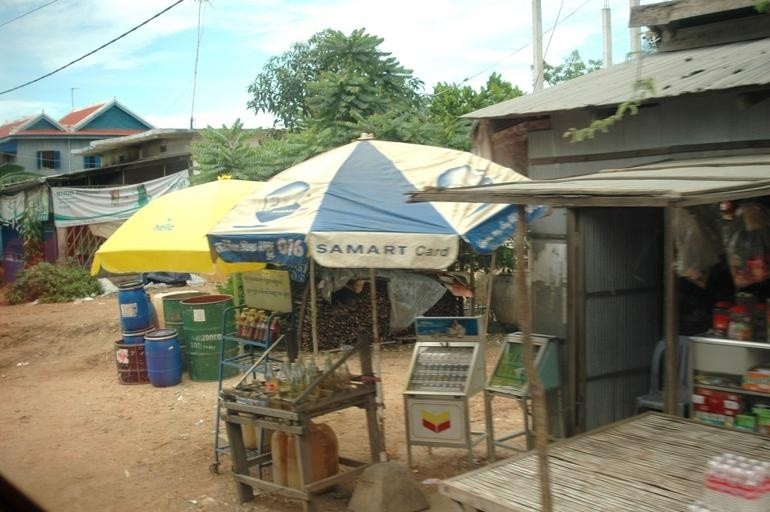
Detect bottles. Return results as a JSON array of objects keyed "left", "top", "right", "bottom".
[
  {"left": 265, "top": 350, "right": 348, "bottom": 409},
  {"left": 702, "top": 453, "right": 770, "bottom": 512},
  {"left": 236, "top": 307, "right": 279, "bottom": 344},
  {"left": 251, "top": 379, "right": 260, "bottom": 399},
  {"left": 712, "top": 303, "right": 727, "bottom": 336}
]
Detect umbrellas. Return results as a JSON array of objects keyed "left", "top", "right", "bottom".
[
  {"left": 205, "top": 132, "right": 550, "bottom": 463},
  {"left": 90, "top": 174, "right": 285, "bottom": 386}
]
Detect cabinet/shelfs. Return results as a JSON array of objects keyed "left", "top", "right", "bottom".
[
  {"left": 216, "top": 303, "right": 386, "bottom": 512},
  {"left": 689, "top": 325, "right": 770, "bottom": 438}
]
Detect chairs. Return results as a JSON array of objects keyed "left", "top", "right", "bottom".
[{"left": 636, "top": 335, "right": 692, "bottom": 418}]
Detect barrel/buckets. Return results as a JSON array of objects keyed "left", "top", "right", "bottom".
[
  {"left": 146, "top": 293, "right": 159, "bottom": 328},
  {"left": 122, "top": 325, "right": 156, "bottom": 344},
  {"left": 286, "top": 423, "right": 338, "bottom": 493},
  {"left": 162, "top": 292, "right": 211, "bottom": 373},
  {"left": 118, "top": 281, "right": 150, "bottom": 329},
  {"left": 271, "top": 430, "right": 287, "bottom": 487},
  {"left": 178, "top": 294, "right": 240, "bottom": 382},
  {"left": 239, "top": 412, "right": 274, "bottom": 449},
  {"left": 144, "top": 329, "right": 182, "bottom": 386}
]
